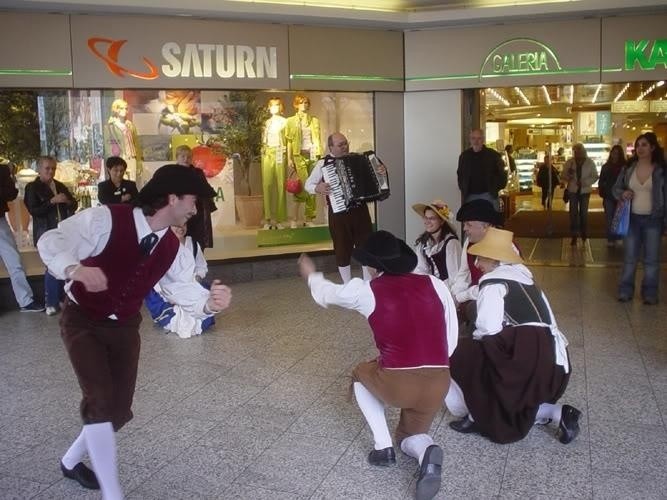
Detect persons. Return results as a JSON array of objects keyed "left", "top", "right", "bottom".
[
  {"left": 33, "top": 164, "right": 232, "bottom": 499},
  {"left": 260, "top": 96, "right": 288, "bottom": 231},
  {"left": 410, "top": 196, "right": 583, "bottom": 445},
  {"left": 283, "top": 94, "right": 324, "bottom": 228},
  {"left": 459, "top": 128, "right": 506, "bottom": 218},
  {"left": 600, "top": 132, "right": 666, "bottom": 305},
  {"left": 103, "top": 99, "right": 143, "bottom": 181},
  {"left": 97, "top": 156, "right": 139, "bottom": 207},
  {"left": 305, "top": 132, "right": 378, "bottom": 283},
  {"left": 507, "top": 144, "right": 517, "bottom": 173},
  {"left": 175, "top": 145, "right": 218, "bottom": 253},
  {"left": 0, "top": 163, "right": 46, "bottom": 313},
  {"left": 144, "top": 222, "right": 217, "bottom": 340},
  {"left": 24, "top": 155, "right": 78, "bottom": 317},
  {"left": 296, "top": 230, "right": 459, "bottom": 500},
  {"left": 559, "top": 143, "right": 598, "bottom": 244},
  {"left": 537, "top": 156, "right": 559, "bottom": 212}
]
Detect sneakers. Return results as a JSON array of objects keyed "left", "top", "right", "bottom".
[
  {"left": 290, "top": 222, "right": 297, "bottom": 229},
  {"left": 571, "top": 236, "right": 577, "bottom": 245},
  {"left": 644, "top": 295, "right": 659, "bottom": 305},
  {"left": 303, "top": 222, "right": 315, "bottom": 227},
  {"left": 20, "top": 300, "right": 46, "bottom": 312},
  {"left": 582, "top": 233, "right": 587, "bottom": 240},
  {"left": 45, "top": 307, "right": 57, "bottom": 316},
  {"left": 618, "top": 296, "right": 633, "bottom": 302}
]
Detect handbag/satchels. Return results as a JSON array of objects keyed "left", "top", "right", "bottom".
[
  {"left": 611, "top": 199, "right": 630, "bottom": 236},
  {"left": 563, "top": 189, "right": 568, "bottom": 203},
  {"left": 285, "top": 168, "right": 301, "bottom": 194}
]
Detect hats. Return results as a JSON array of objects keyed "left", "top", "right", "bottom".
[
  {"left": 411, "top": 200, "right": 456, "bottom": 234},
  {"left": 456, "top": 199, "right": 505, "bottom": 227},
  {"left": 350, "top": 230, "right": 417, "bottom": 275},
  {"left": 467, "top": 226, "right": 525, "bottom": 264},
  {"left": 140, "top": 165, "right": 208, "bottom": 199}
]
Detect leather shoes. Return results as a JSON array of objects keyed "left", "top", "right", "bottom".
[
  {"left": 559, "top": 405, "right": 581, "bottom": 444},
  {"left": 413, "top": 446, "right": 443, "bottom": 500},
  {"left": 449, "top": 415, "right": 483, "bottom": 433},
  {"left": 368, "top": 446, "right": 396, "bottom": 466},
  {"left": 60, "top": 459, "right": 100, "bottom": 488}
]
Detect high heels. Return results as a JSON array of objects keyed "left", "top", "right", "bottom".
[
  {"left": 275, "top": 223, "right": 284, "bottom": 230},
  {"left": 263, "top": 223, "right": 272, "bottom": 230}
]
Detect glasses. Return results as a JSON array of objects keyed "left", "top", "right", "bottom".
[
  {"left": 422, "top": 215, "right": 440, "bottom": 223},
  {"left": 331, "top": 141, "right": 348, "bottom": 148}
]
